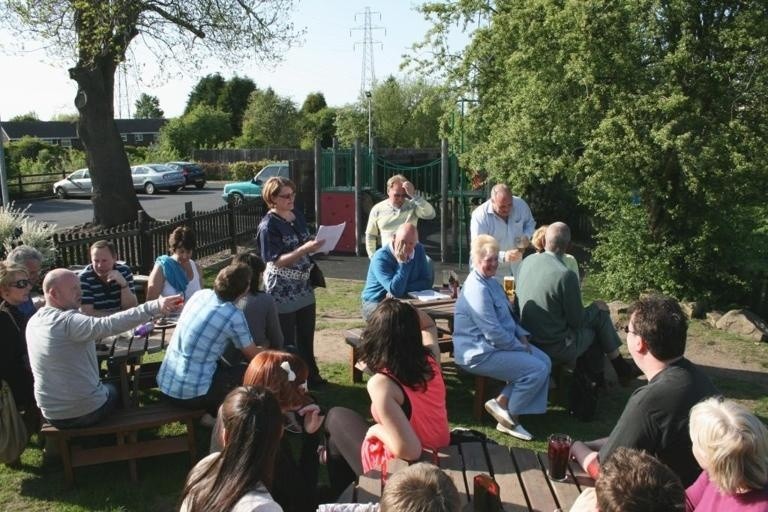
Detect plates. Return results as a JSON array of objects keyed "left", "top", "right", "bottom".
[{"left": 150, "top": 321, "right": 178, "bottom": 327}]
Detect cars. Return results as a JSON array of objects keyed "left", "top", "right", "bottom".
[
  {"left": 53, "top": 168, "right": 93, "bottom": 198},
  {"left": 130, "top": 163, "right": 185, "bottom": 194}
]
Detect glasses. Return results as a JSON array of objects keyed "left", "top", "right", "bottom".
[
  {"left": 8, "top": 279, "right": 31, "bottom": 288},
  {"left": 276, "top": 191, "right": 296, "bottom": 198}
]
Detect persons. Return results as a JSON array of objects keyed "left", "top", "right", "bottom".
[
  {"left": 359, "top": 175, "right": 436, "bottom": 314},
  {"left": 569, "top": 294, "right": 766, "bottom": 511},
  {"left": 180, "top": 297, "right": 461, "bottom": 512},
  {"left": 256, "top": 176, "right": 328, "bottom": 387},
  {"left": 451, "top": 183, "right": 633, "bottom": 440},
  {"left": 157, "top": 252, "right": 286, "bottom": 428},
  {"left": 2, "top": 226, "right": 203, "bottom": 445}
]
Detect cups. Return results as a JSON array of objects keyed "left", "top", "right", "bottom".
[
  {"left": 442, "top": 270, "right": 450, "bottom": 288},
  {"left": 547, "top": 433, "right": 571, "bottom": 480},
  {"left": 171, "top": 292, "right": 184, "bottom": 310},
  {"left": 503, "top": 273, "right": 515, "bottom": 297},
  {"left": 515, "top": 234, "right": 526, "bottom": 255}
]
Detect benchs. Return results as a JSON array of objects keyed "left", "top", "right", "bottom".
[
  {"left": 347, "top": 332, "right": 444, "bottom": 383},
  {"left": 40, "top": 402, "right": 208, "bottom": 487},
  {"left": 453, "top": 347, "right": 600, "bottom": 422}
]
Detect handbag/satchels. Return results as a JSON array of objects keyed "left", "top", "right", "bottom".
[
  {"left": 1, "top": 379, "right": 28, "bottom": 464},
  {"left": 451, "top": 428, "right": 498, "bottom": 445},
  {"left": 556, "top": 370, "right": 599, "bottom": 423},
  {"left": 310, "top": 261, "right": 326, "bottom": 287}
]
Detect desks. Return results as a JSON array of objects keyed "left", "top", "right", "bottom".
[
  {"left": 334, "top": 442, "right": 595, "bottom": 512},
  {"left": 90, "top": 305, "right": 180, "bottom": 408},
  {"left": 393, "top": 286, "right": 515, "bottom": 358}
]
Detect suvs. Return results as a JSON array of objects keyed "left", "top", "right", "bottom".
[
  {"left": 164, "top": 160, "right": 206, "bottom": 191},
  {"left": 222, "top": 163, "right": 289, "bottom": 207}
]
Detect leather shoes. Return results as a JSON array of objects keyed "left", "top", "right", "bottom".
[
  {"left": 620, "top": 357, "right": 642, "bottom": 387},
  {"left": 496, "top": 422, "right": 534, "bottom": 440},
  {"left": 199, "top": 413, "right": 217, "bottom": 427},
  {"left": 484, "top": 399, "right": 515, "bottom": 429}
]
